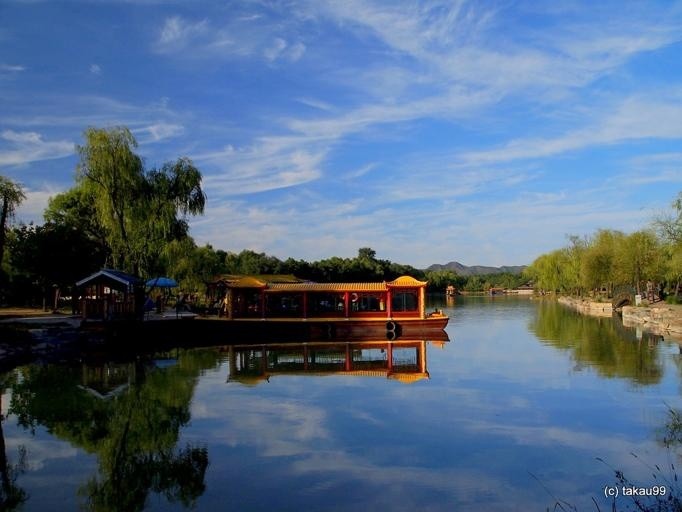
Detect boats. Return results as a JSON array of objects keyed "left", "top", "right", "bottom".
[
  {"left": 192, "top": 275, "right": 448, "bottom": 331},
  {"left": 191, "top": 330, "right": 450, "bottom": 387}
]
